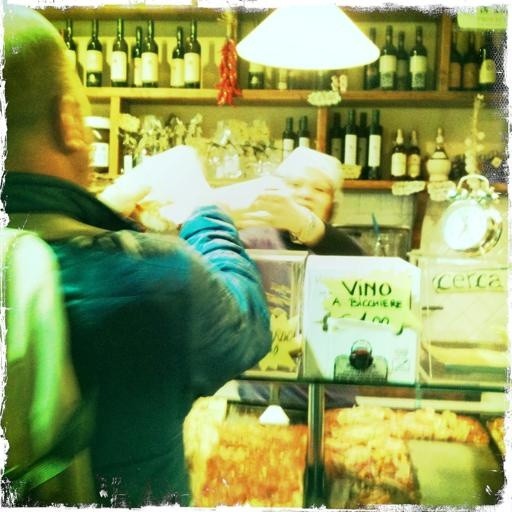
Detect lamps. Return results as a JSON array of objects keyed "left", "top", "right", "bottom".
[{"left": 235, "top": 6, "right": 381, "bottom": 72}]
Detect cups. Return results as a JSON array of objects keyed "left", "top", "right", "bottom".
[{"left": 349, "top": 339, "right": 374, "bottom": 370}]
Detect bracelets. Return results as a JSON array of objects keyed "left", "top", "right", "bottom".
[{"left": 292, "top": 206, "right": 317, "bottom": 240}]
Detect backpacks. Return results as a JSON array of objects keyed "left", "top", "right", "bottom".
[{"left": 2, "top": 212, "right": 96, "bottom": 503}]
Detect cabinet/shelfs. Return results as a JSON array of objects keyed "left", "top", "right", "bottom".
[{"left": 38, "top": 6, "right": 509, "bottom": 508}]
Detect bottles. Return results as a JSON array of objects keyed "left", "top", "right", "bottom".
[
  {"left": 391, "top": 127, "right": 422, "bottom": 183},
  {"left": 363, "top": 22, "right": 429, "bottom": 92},
  {"left": 281, "top": 116, "right": 311, "bottom": 162},
  {"left": 246, "top": 64, "right": 350, "bottom": 93},
  {"left": 153, "top": 111, "right": 175, "bottom": 152},
  {"left": 327, "top": 108, "right": 383, "bottom": 180},
  {"left": 63, "top": 17, "right": 202, "bottom": 90},
  {"left": 424, "top": 126, "right": 454, "bottom": 182},
  {"left": 90, "top": 115, "right": 110, "bottom": 174},
  {"left": 449, "top": 31, "right": 499, "bottom": 91}
]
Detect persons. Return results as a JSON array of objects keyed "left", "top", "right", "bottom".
[
  {"left": 228, "top": 142, "right": 366, "bottom": 430},
  {"left": 4, "top": 4, "right": 271, "bottom": 508}
]
